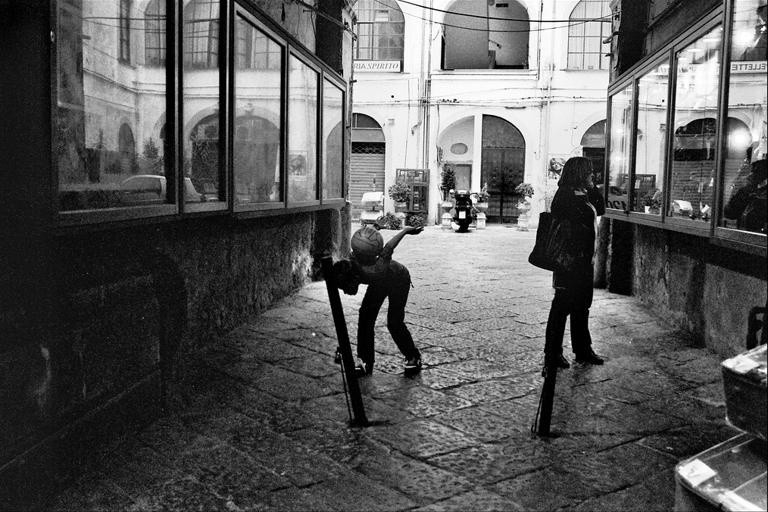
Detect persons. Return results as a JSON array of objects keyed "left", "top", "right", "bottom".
[
  {"left": 722, "top": 156, "right": 768, "bottom": 231},
  {"left": 333, "top": 222, "right": 422, "bottom": 376},
  {"left": 544, "top": 155, "right": 606, "bottom": 370}
]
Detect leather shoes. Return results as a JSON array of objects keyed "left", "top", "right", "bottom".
[
  {"left": 577, "top": 346, "right": 603, "bottom": 364},
  {"left": 543, "top": 344, "right": 570, "bottom": 369}
]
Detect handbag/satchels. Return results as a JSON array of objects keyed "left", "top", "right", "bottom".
[{"left": 529, "top": 212, "right": 588, "bottom": 273}]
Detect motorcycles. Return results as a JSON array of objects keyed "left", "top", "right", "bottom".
[{"left": 448, "top": 189, "right": 479, "bottom": 234}]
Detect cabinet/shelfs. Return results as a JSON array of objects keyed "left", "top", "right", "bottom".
[{"left": 395, "top": 168, "right": 430, "bottom": 226}]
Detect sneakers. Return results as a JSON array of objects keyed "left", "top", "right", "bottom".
[
  {"left": 355, "top": 355, "right": 373, "bottom": 376},
  {"left": 404, "top": 350, "right": 421, "bottom": 375}
]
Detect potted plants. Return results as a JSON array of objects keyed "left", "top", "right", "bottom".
[
  {"left": 474, "top": 182, "right": 490, "bottom": 213},
  {"left": 440, "top": 159, "right": 457, "bottom": 213},
  {"left": 388, "top": 181, "right": 411, "bottom": 213},
  {"left": 515, "top": 182, "right": 535, "bottom": 214}
]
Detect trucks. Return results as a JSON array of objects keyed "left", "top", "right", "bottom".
[{"left": 117, "top": 175, "right": 206, "bottom": 204}]
